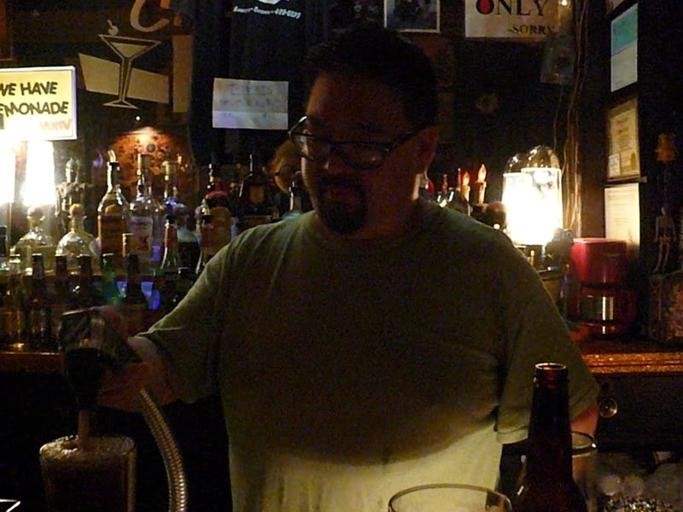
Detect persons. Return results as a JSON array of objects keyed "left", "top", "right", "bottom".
[{"left": 63, "top": 26, "right": 602, "bottom": 510}]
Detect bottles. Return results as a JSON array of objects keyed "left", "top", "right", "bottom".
[
  {"left": 641, "top": 127, "right": 683, "bottom": 353},
  {"left": 419, "top": 165, "right": 490, "bottom": 224},
  {"left": 514, "top": 362, "right": 588, "bottom": 512},
  {"left": 0, "top": 150, "right": 316, "bottom": 356},
  {"left": 571, "top": 238, "right": 640, "bottom": 338}
]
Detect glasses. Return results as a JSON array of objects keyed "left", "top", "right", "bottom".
[{"left": 288, "top": 115, "right": 421, "bottom": 171}]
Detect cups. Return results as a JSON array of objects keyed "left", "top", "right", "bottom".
[
  {"left": 37, "top": 432, "right": 138, "bottom": 512},
  {"left": 570, "top": 429, "right": 601, "bottom": 512}
]
